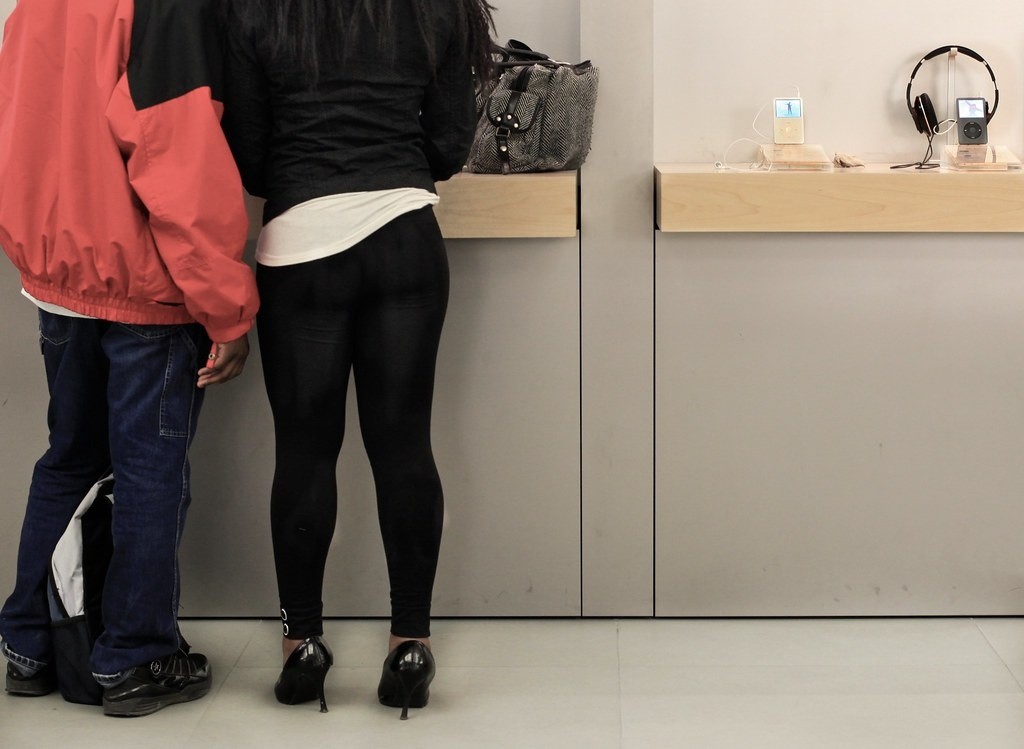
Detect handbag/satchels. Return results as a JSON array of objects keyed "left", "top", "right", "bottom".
[{"left": 463, "top": 38, "right": 598, "bottom": 174}]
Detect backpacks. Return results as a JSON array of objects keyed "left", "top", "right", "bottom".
[{"left": 43, "top": 472, "right": 116, "bottom": 706}]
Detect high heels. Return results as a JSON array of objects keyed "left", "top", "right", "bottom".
[
  {"left": 377, "top": 640, "right": 436, "bottom": 720},
  {"left": 274, "top": 635, "right": 333, "bottom": 714}
]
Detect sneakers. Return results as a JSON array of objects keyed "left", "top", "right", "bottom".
[
  {"left": 5, "top": 659, "right": 60, "bottom": 695},
  {"left": 102, "top": 653, "right": 212, "bottom": 718}
]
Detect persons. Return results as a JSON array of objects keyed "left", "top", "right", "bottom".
[
  {"left": 0, "top": 0, "right": 258, "bottom": 715},
  {"left": 211, "top": 0, "right": 479, "bottom": 720}
]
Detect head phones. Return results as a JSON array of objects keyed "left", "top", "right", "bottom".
[{"left": 906, "top": 44, "right": 999, "bottom": 136}]
